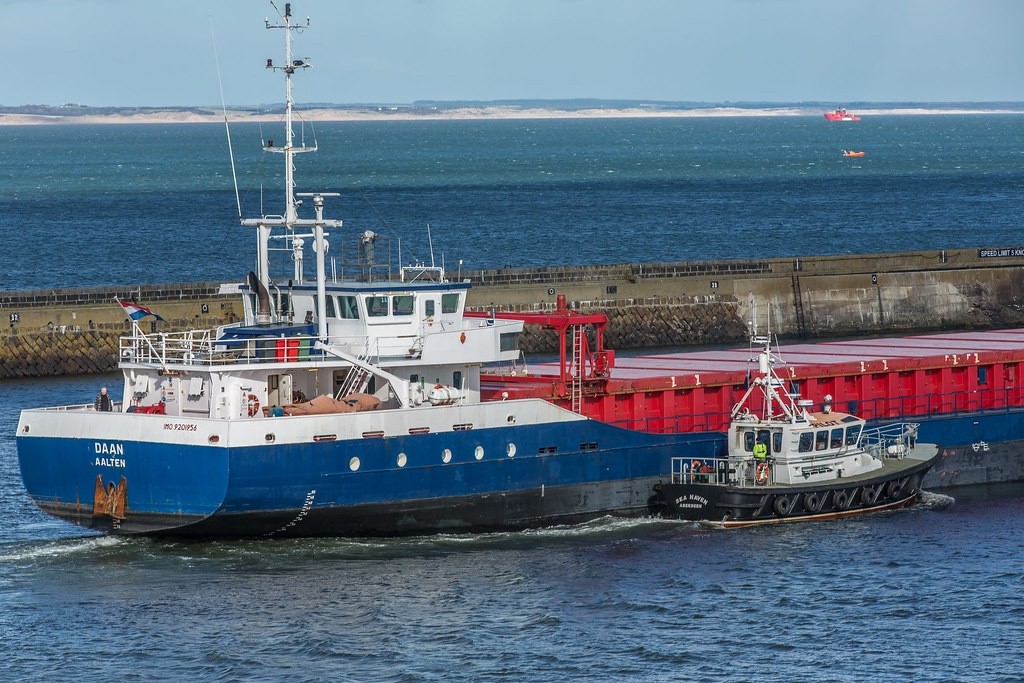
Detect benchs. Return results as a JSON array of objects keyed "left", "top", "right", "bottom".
[{"left": 672, "top": 472, "right": 717, "bottom": 484}]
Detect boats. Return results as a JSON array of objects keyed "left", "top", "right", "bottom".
[{"left": 15, "top": 0, "right": 1024, "bottom": 539}]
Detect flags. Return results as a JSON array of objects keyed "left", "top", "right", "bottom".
[{"left": 120, "top": 301, "right": 166, "bottom": 323}]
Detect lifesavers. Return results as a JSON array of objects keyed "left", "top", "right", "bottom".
[
  {"left": 755, "top": 463, "right": 769, "bottom": 485},
  {"left": 247, "top": 394, "right": 259, "bottom": 416},
  {"left": 804, "top": 492, "right": 820, "bottom": 513},
  {"left": 887, "top": 482, "right": 901, "bottom": 500},
  {"left": 861, "top": 488, "right": 876, "bottom": 506},
  {"left": 833, "top": 492, "right": 848, "bottom": 510},
  {"left": 690, "top": 460, "right": 702, "bottom": 482},
  {"left": 774, "top": 496, "right": 793, "bottom": 517}
]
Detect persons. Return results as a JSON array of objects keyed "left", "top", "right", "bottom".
[
  {"left": 753, "top": 437, "right": 767, "bottom": 472},
  {"left": 94, "top": 387, "right": 112, "bottom": 412}
]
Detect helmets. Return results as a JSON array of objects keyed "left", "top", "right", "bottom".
[{"left": 756, "top": 437, "right": 762, "bottom": 441}]
